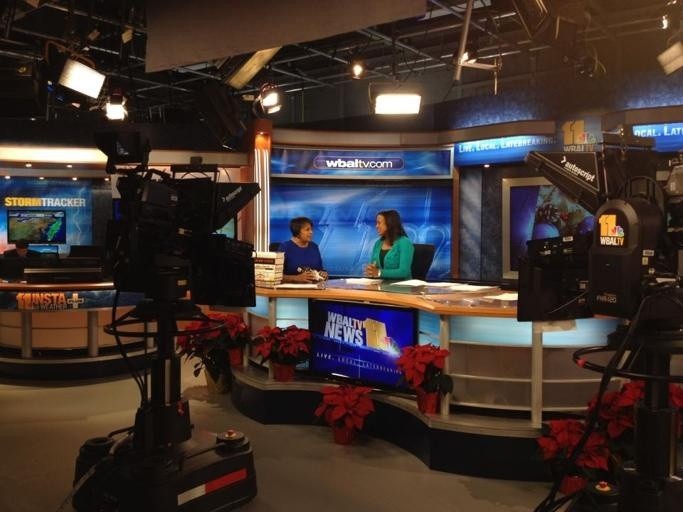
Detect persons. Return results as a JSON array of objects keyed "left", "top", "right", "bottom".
[
  {"left": 362, "top": 209, "right": 415, "bottom": 280},
  {"left": 2, "top": 239, "right": 41, "bottom": 257},
  {"left": 275, "top": 216, "right": 330, "bottom": 284}
]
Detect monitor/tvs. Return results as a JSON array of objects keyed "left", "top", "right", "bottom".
[
  {"left": 27, "top": 246, "right": 59, "bottom": 255},
  {"left": 8, "top": 210, "right": 66, "bottom": 243},
  {"left": 308, "top": 298, "right": 418, "bottom": 396},
  {"left": 24, "top": 266, "right": 103, "bottom": 284}
]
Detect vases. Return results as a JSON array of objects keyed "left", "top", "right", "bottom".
[
  {"left": 226, "top": 347, "right": 243, "bottom": 366},
  {"left": 415, "top": 393, "right": 439, "bottom": 414},
  {"left": 271, "top": 362, "right": 298, "bottom": 381},
  {"left": 556, "top": 476, "right": 589, "bottom": 495},
  {"left": 331, "top": 428, "right": 354, "bottom": 445},
  {"left": 204, "top": 368, "right": 230, "bottom": 395}
]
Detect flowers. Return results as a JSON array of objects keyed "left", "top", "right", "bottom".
[
  {"left": 536, "top": 421, "right": 612, "bottom": 476},
  {"left": 251, "top": 324, "right": 312, "bottom": 366},
  {"left": 396, "top": 343, "right": 455, "bottom": 395},
  {"left": 175, "top": 312, "right": 249, "bottom": 382},
  {"left": 316, "top": 385, "right": 374, "bottom": 429},
  {"left": 588, "top": 380, "right": 682, "bottom": 439}
]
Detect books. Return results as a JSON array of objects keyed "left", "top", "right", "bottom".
[
  {"left": 252, "top": 250, "right": 286, "bottom": 288},
  {"left": 272, "top": 283, "right": 324, "bottom": 290}
]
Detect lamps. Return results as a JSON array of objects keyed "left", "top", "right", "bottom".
[
  {"left": 257, "top": 83, "right": 282, "bottom": 118},
  {"left": 367, "top": 31, "right": 426, "bottom": 116}
]
